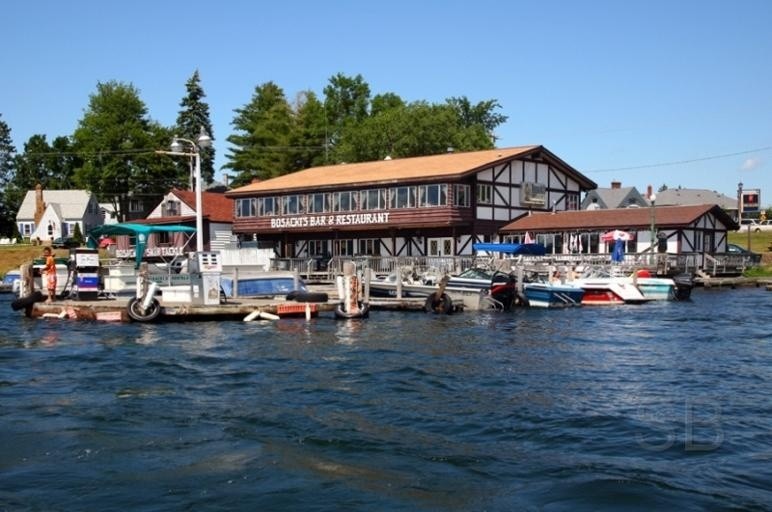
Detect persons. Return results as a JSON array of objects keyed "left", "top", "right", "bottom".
[
  {"left": 40, "top": 247, "right": 57, "bottom": 304},
  {"left": 525, "top": 261, "right": 584, "bottom": 286},
  {"left": 21, "top": 256, "right": 34, "bottom": 297}
]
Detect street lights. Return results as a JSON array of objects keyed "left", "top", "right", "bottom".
[
  {"left": 168, "top": 122, "right": 216, "bottom": 259},
  {"left": 650, "top": 192, "right": 657, "bottom": 267}
]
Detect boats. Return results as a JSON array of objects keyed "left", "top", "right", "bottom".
[{"left": 2, "top": 206, "right": 698, "bottom": 323}]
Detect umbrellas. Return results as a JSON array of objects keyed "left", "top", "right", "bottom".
[
  {"left": 601, "top": 229, "right": 634, "bottom": 242},
  {"left": 612, "top": 237, "right": 624, "bottom": 263}
]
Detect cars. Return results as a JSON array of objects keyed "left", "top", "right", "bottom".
[{"left": 734, "top": 215, "right": 772, "bottom": 235}]
